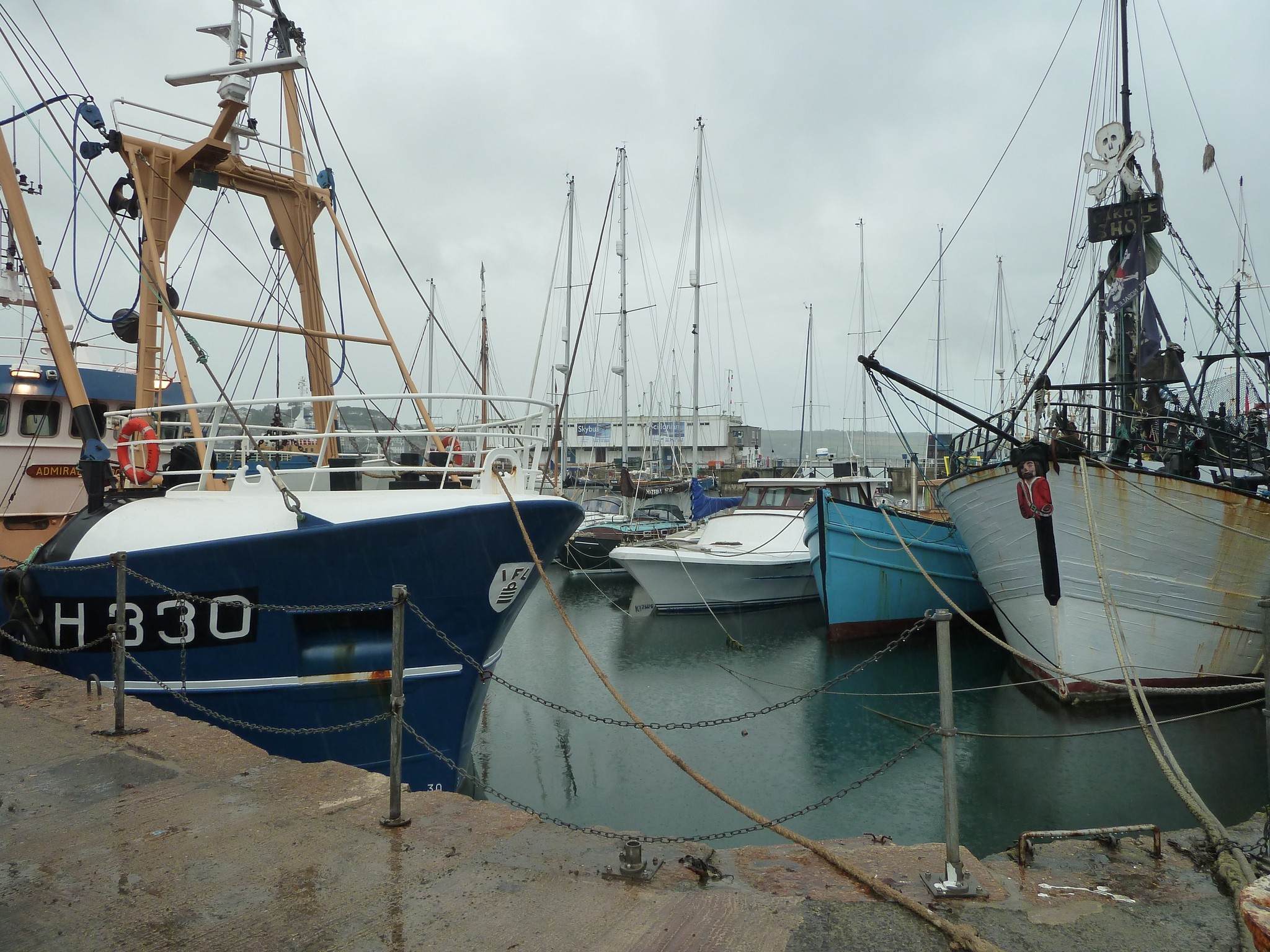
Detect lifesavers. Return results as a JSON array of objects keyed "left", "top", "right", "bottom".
[
  {"left": 440, "top": 436, "right": 464, "bottom": 467},
  {"left": 115, "top": 419, "right": 161, "bottom": 485}
]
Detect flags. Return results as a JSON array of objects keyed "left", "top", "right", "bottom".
[
  {"left": 1103, "top": 223, "right": 1146, "bottom": 314},
  {"left": 1141, "top": 283, "right": 1162, "bottom": 344}
]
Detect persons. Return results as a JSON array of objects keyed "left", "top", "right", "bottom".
[
  {"left": 165, "top": 415, "right": 176, "bottom": 439},
  {"left": 1017, "top": 453, "right": 1054, "bottom": 519},
  {"left": 787, "top": 494, "right": 803, "bottom": 507}
]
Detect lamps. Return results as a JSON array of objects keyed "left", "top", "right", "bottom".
[
  {"left": 9, "top": 364, "right": 41, "bottom": 379},
  {"left": 154, "top": 374, "right": 172, "bottom": 390}
]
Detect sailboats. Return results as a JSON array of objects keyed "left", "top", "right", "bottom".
[
  {"left": 857, "top": 0, "right": 1270, "bottom": 706},
  {"left": 0, "top": 1, "right": 586, "bottom": 795},
  {"left": 0, "top": 108, "right": 994, "bottom": 633}
]
